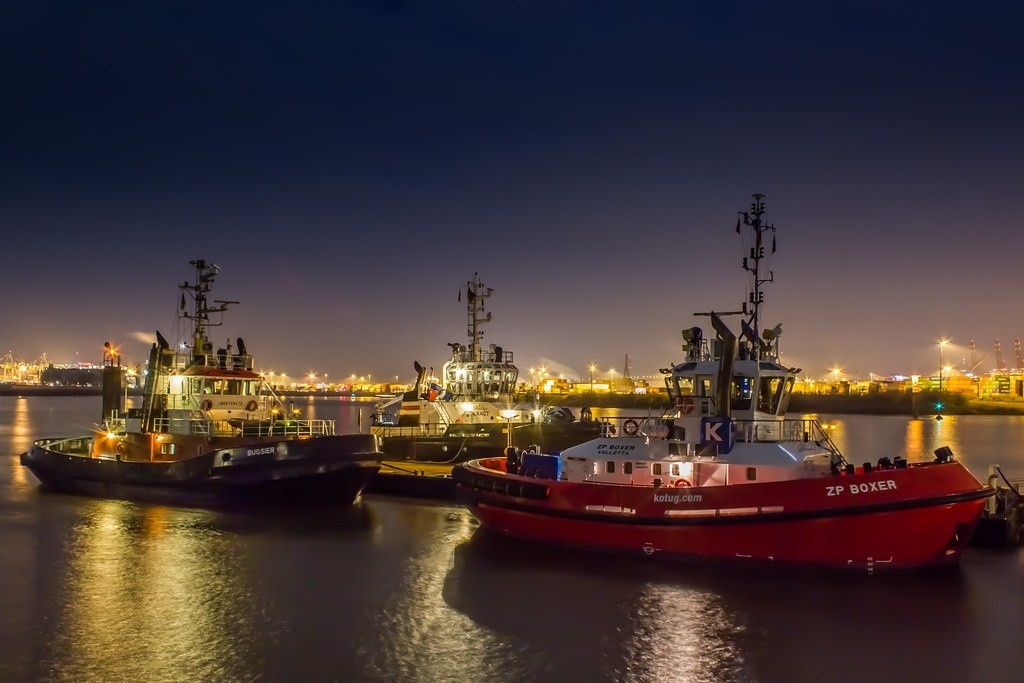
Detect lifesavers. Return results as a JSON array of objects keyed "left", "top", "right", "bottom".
[
  {"left": 675, "top": 478, "right": 691, "bottom": 487},
  {"left": 247, "top": 400, "right": 258, "bottom": 411},
  {"left": 115, "top": 442, "right": 125, "bottom": 456},
  {"left": 201, "top": 399, "right": 213, "bottom": 411},
  {"left": 678, "top": 398, "right": 694, "bottom": 415},
  {"left": 623, "top": 419, "right": 639, "bottom": 435}
]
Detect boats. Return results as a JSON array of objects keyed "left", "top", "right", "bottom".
[
  {"left": 101, "top": 258, "right": 337, "bottom": 441},
  {"left": 450, "top": 194, "right": 997, "bottom": 577},
  {"left": 20, "top": 430, "right": 385, "bottom": 510},
  {"left": 372, "top": 269, "right": 616, "bottom": 487},
  {"left": 966, "top": 461, "right": 1024, "bottom": 551}
]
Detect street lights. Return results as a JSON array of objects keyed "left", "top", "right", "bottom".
[
  {"left": 938, "top": 337, "right": 951, "bottom": 392},
  {"left": 590, "top": 363, "right": 596, "bottom": 391}
]
